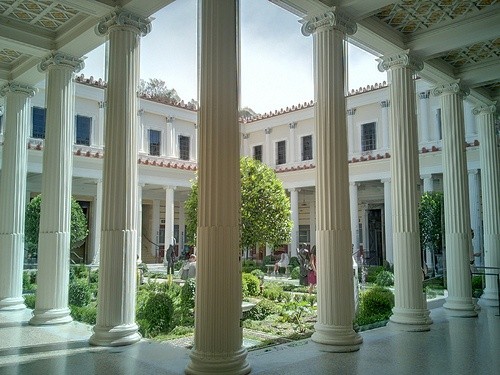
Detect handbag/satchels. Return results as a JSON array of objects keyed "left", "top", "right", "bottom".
[{"left": 305, "top": 263, "right": 316, "bottom": 271}]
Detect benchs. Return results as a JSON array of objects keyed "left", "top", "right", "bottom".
[{"left": 265, "top": 264, "right": 293, "bottom": 275}]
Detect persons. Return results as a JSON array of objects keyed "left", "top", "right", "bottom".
[
  {"left": 298, "top": 241, "right": 309, "bottom": 286},
  {"left": 166, "top": 243, "right": 175, "bottom": 275},
  {"left": 472, "top": 227, "right": 481, "bottom": 277},
  {"left": 181, "top": 254, "right": 197, "bottom": 277},
  {"left": 306, "top": 244, "right": 318, "bottom": 297},
  {"left": 271, "top": 247, "right": 288, "bottom": 277}
]
